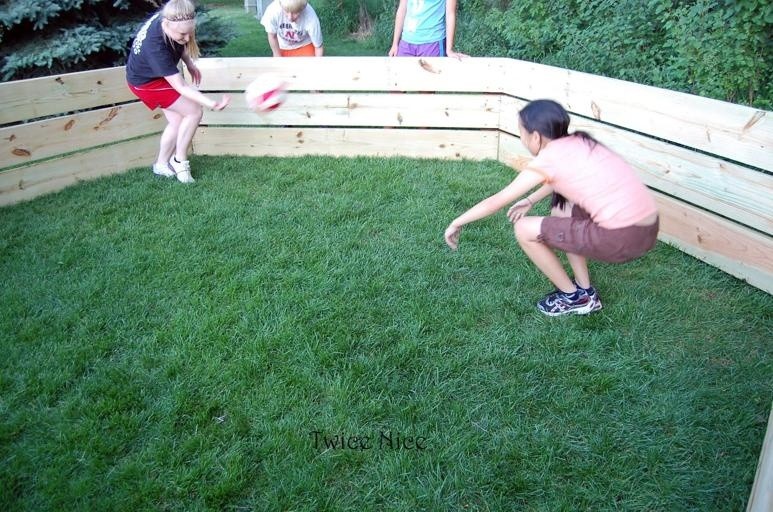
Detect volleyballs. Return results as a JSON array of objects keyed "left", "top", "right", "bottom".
[{"left": 244, "top": 77, "right": 288, "bottom": 114}]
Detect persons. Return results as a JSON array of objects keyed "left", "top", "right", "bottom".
[
  {"left": 389, "top": 0, "right": 471, "bottom": 59},
  {"left": 445, "top": 100, "right": 661, "bottom": 316},
  {"left": 126, "top": 0, "right": 232, "bottom": 183},
  {"left": 260, "top": 0, "right": 324, "bottom": 93}
]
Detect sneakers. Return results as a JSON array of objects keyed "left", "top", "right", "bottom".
[
  {"left": 537, "top": 281, "right": 602, "bottom": 316},
  {"left": 153, "top": 153, "right": 195, "bottom": 184}
]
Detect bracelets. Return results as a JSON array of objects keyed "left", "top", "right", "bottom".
[
  {"left": 525, "top": 197, "right": 534, "bottom": 207},
  {"left": 210, "top": 102, "right": 220, "bottom": 112}
]
